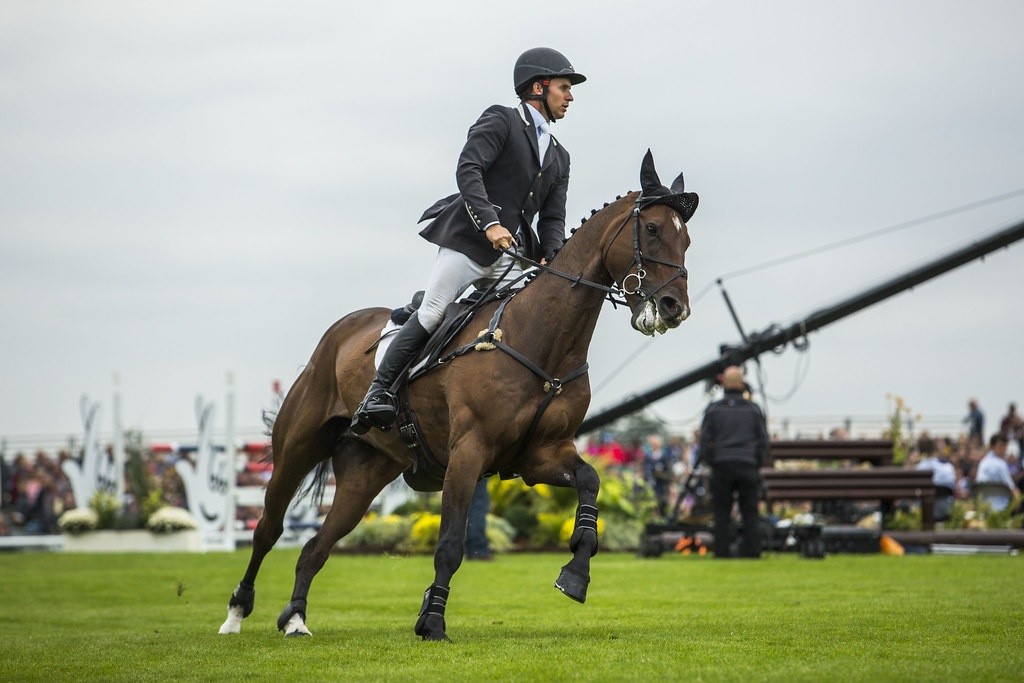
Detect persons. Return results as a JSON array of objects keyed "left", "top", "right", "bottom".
[
  {"left": 961, "top": 401, "right": 1022, "bottom": 523},
  {"left": 697, "top": 365, "right": 771, "bottom": 558},
  {"left": 791, "top": 500, "right": 815, "bottom": 527},
  {"left": 901, "top": 431, "right": 958, "bottom": 523},
  {"left": 644, "top": 428, "right": 702, "bottom": 523},
  {"left": 353, "top": 45, "right": 588, "bottom": 427},
  {"left": 0, "top": 442, "right": 210, "bottom": 546}
]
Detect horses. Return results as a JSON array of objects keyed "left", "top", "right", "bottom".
[{"left": 215, "top": 146, "right": 701, "bottom": 640}]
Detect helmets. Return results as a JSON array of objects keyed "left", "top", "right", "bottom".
[{"left": 514, "top": 47, "right": 587, "bottom": 91}]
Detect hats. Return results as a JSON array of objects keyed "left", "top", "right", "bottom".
[{"left": 724, "top": 365, "right": 744, "bottom": 390}]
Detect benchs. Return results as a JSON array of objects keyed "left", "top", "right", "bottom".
[{"left": 693, "top": 439, "right": 937, "bottom": 529}]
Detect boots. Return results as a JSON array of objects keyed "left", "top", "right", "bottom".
[{"left": 350, "top": 309, "right": 429, "bottom": 435}]
